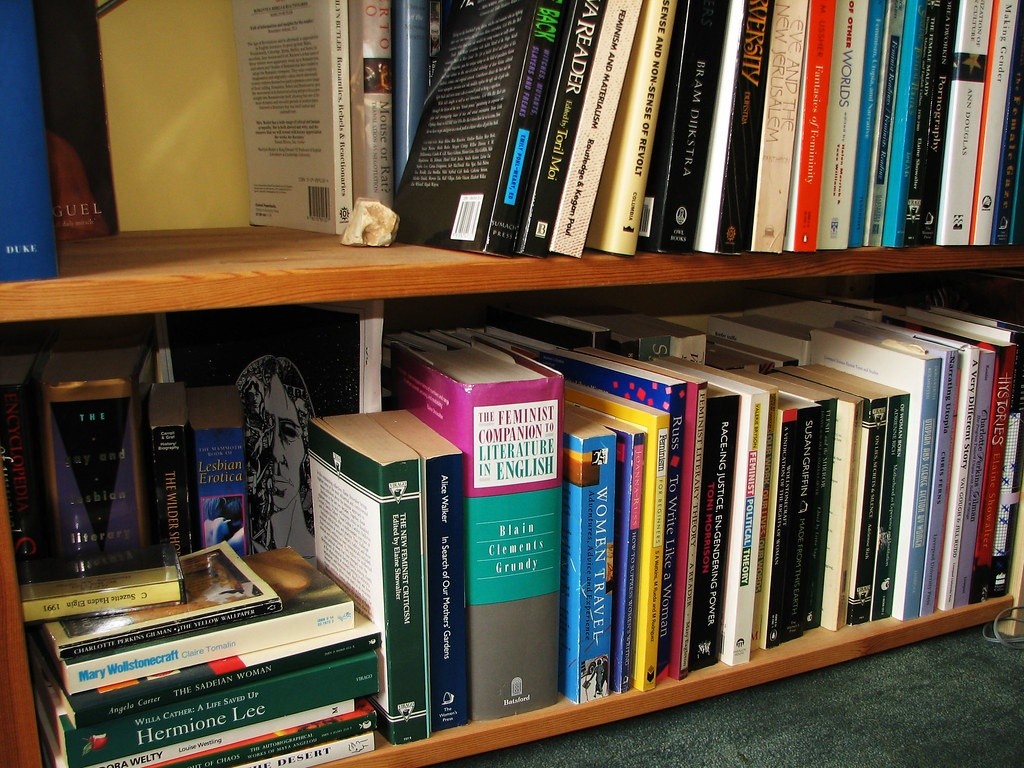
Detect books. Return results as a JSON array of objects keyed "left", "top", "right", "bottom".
[
  {"left": 309, "top": 297, "right": 1024, "bottom": 746},
  {"left": 233, "top": 0, "right": 1024, "bottom": 259},
  {"left": 0, "top": 299, "right": 384, "bottom": 768},
  {"left": 0, "top": 1, "right": 119, "bottom": 282}
]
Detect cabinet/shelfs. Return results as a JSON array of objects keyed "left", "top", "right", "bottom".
[{"left": 0, "top": 227, "right": 1024, "bottom": 768}]
